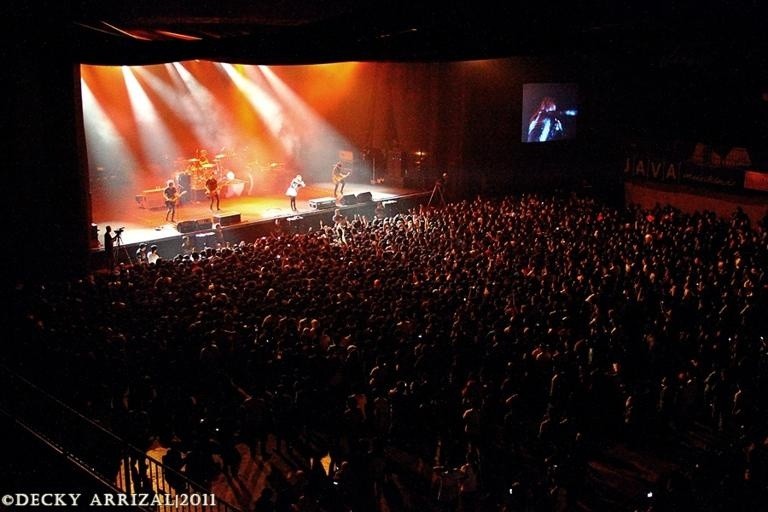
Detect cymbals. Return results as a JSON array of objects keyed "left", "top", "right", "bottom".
[
  {"left": 213, "top": 160, "right": 222, "bottom": 162},
  {"left": 188, "top": 158, "right": 200, "bottom": 162},
  {"left": 215, "top": 155, "right": 224, "bottom": 158}
]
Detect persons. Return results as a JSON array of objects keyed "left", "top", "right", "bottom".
[
  {"left": 332, "top": 162, "right": 346, "bottom": 197},
  {"left": 176, "top": 170, "right": 193, "bottom": 209},
  {"left": 163, "top": 180, "right": 179, "bottom": 223},
  {"left": 197, "top": 149, "right": 214, "bottom": 180},
  {"left": 527, "top": 96, "right": 563, "bottom": 143},
  {"left": 285, "top": 174, "right": 305, "bottom": 211},
  {"left": 10, "top": 186, "right": 766, "bottom": 512},
  {"left": 205, "top": 173, "right": 222, "bottom": 211}
]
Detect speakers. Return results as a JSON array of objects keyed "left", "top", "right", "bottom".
[
  {"left": 113, "top": 235, "right": 134, "bottom": 267},
  {"left": 357, "top": 192, "right": 372, "bottom": 203},
  {"left": 176, "top": 218, "right": 212, "bottom": 233},
  {"left": 214, "top": 213, "right": 241, "bottom": 226},
  {"left": 340, "top": 195, "right": 358, "bottom": 205}
]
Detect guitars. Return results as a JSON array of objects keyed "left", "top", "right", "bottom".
[
  {"left": 164, "top": 190, "right": 187, "bottom": 208},
  {"left": 332, "top": 171, "right": 350, "bottom": 184},
  {"left": 205, "top": 185, "right": 219, "bottom": 197}
]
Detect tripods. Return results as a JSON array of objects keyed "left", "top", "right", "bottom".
[{"left": 426, "top": 182, "right": 446, "bottom": 208}]
